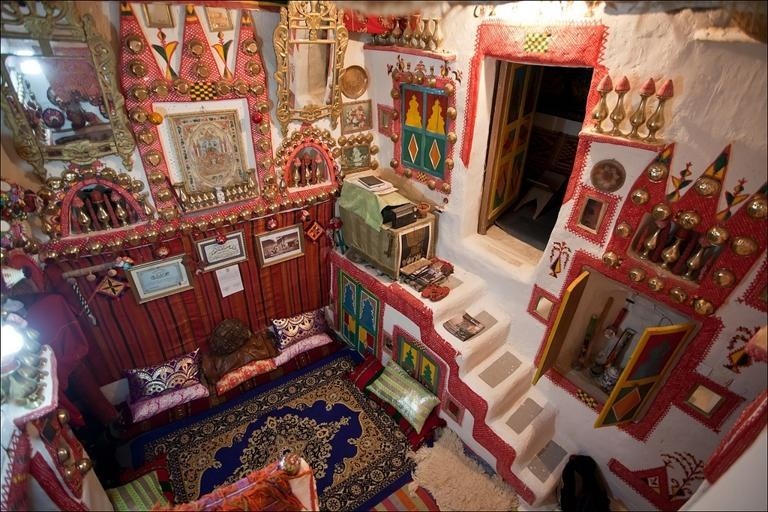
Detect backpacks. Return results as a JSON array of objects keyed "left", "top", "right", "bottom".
[{"left": 560, "top": 454, "right": 611, "bottom": 512}]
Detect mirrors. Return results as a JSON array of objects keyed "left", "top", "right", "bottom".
[
  {"left": 1, "top": 1, "right": 136, "bottom": 183},
  {"left": 273, "top": 0, "right": 349, "bottom": 141}
]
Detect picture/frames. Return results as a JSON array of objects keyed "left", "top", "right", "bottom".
[
  {"left": 341, "top": 144, "right": 371, "bottom": 172},
  {"left": 193, "top": 229, "right": 248, "bottom": 276},
  {"left": 253, "top": 222, "right": 305, "bottom": 268},
  {"left": 340, "top": 99, "right": 374, "bottom": 134},
  {"left": 123, "top": 253, "right": 194, "bottom": 305}
]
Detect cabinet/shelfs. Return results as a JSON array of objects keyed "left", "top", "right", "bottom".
[{"left": 534, "top": 250, "right": 718, "bottom": 443}]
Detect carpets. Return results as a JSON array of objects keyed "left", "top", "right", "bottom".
[{"left": 127, "top": 347, "right": 421, "bottom": 512}]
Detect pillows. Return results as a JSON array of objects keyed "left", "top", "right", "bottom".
[
  {"left": 106, "top": 451, "right": 174, "bottom": 512},
  {"left": 341, "top": 354, "right": 445, "bottom": 454},
  {"left": 123, "top": 306, "right": 333, "bottom": 422}
]
